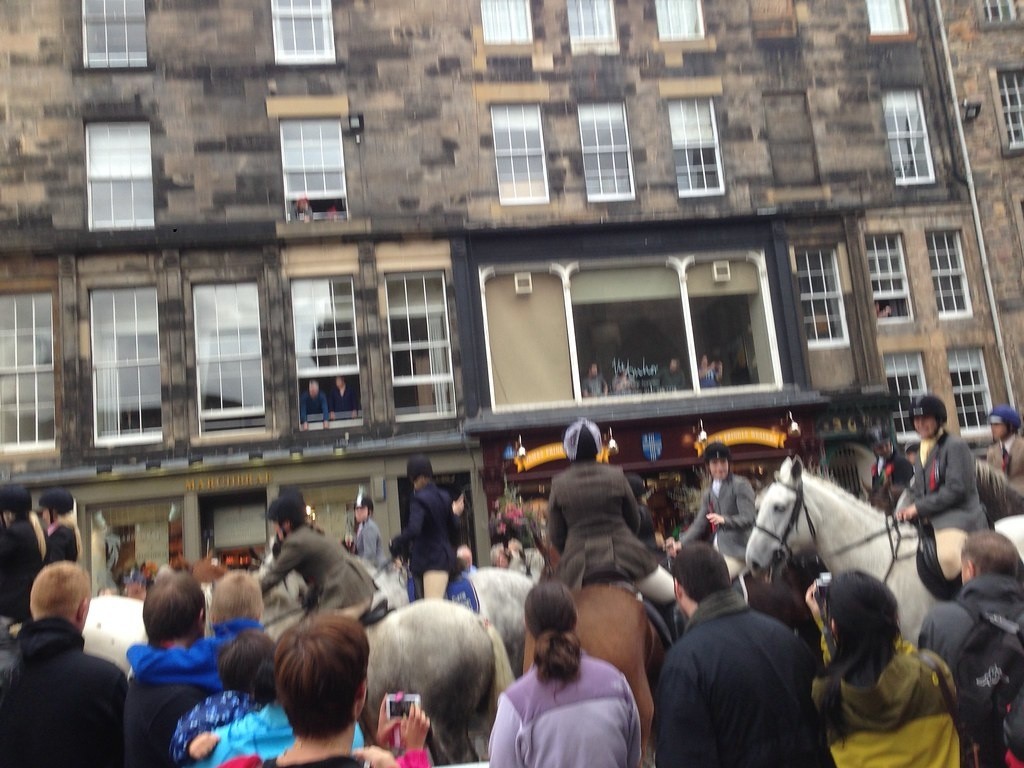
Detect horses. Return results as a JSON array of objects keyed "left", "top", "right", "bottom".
[
  {"left": 523, "top": 583, "right": 666, "bottom": 768},
  {"left": 259, "top": 535, "right": 539, "bottom": 680},
  {"left": 746, "top": 454, "right": 1024, "bottom": 666},
  {"left": 77, "top": 595, "right": 515, "bottom": 768}
]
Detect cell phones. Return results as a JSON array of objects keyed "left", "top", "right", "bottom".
[{"left": 386, "top": 693, "right": 421, "bottom": 721}]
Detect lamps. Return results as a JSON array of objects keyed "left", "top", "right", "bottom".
[
  {"left": 780, "top": 410, "right": 801, "bottom": 439},
  {"left": 603, "top": 426, "right": 618, "bottom": 457},
  {"left": 146, "top": 458, "right": 162, "bottom": 473},
  {"left": 188, "top": 454, "right": 204, "bottom": 470},
  {"left": 961, "top": 97, "right": 981, "bottom": 123},
  {"left": 248, "top": 451, "right": 264, "bottom": 466},
  {"left": 692, "top": 418, "right": 707, "bottom": 444},
  {"left": 514, "top": 433, "right": 526, "bottom": 458},
  {"left": 332, "top": 445, "right": 346, "bottom": 458},
  {"left": 96, "top": 464, "right": 113, "bottom": 481},
  {"left": 289, "top": 448, "right": 304, "bottom": 463}
]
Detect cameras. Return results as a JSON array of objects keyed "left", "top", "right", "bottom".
[{"left": 815, "top": 572, "right": 834, "bottom": 600}]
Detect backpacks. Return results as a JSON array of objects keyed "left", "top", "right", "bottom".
[{"left": 951, "top": 599, "right": 1023, "bottom": 748}]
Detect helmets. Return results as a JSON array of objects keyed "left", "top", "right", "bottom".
[
  {"left": 629, "top": 476, "right": 644, "bottom": 495},
  {"left": 354, "top": 498, "right": 374, "bottom": 510},
  {"left": 0, "top": 486, "right": 32, "bottom": 512},
  {"left": 909, "top": 396, "right": 946, "bottom": 428},
  {"left": 406, "top": 458, "right": 432, "bottom": 479},
  {"left": 563, "top": 417, "right": 601, "bottom": 458},
  {"left": 267, "top": 496, "right": 304, "bottom": 524},
  {"left": 703, "top": 443, "right": 730, "bottom": 462},
  {"left": 867, "top": 426, "right": 891, "bottom": 448},
  {"left": 39, "top": 488, "right": 74, "bottom": 514},
  {"left": 989, "top": 407, "right": 1020, "bottom": 430}
]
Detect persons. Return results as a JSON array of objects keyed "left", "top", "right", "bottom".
[
  {"left": 116, "top": 572, "right": 208, "bottom": 768},
  {"left": 346, "top": 492, "right": 388, "bottom": 571},
  {"left": 216, "top": 613, "right": 434, "bottom": 768},
  {"left": 0, "top": 560, "right": 128, "bottom": 768},
  {"left": 299, "top": 377, "right": 359, "bottom": 430},
  {"left": 168, "top": 629, "right": 363, "bottom": 768},
  {"left": 94, "top": 553, "right": 227, "bottom": 600},
  {"left": 0, "top": 484, "right": 47, "bottom": 624},
  {"left": 127, "top": 569, "right": 265, "bottom": 692},
  {"left": 484, "top": 582, "right": 644, "bottom": 768},
  {"left": 41, "top": 489, "right": 84, "bottom": 565},
  {"left": 257, "top": 492, "right": 377, "bottom": 623},
  {"left": 651, "top": 545, "right": 830, "bottom": 767},
  {"left": 291, "top": 198, "right": 314, "bottom": 225},
  {"left": 653, "top": 436, "right": 761, "bottom": 587},
  {"left": 390, "top": 456, "right": 460, "bottom": 603},
  {"left": 544, "top": 420, "right": 683, "bottom": 637},
  {"left": 580, "top": 347, "right": 737, "bottom": 398},
  {"left": 806, "top": 569, "right": 961, "bottom": 768},
  {"left": 914, "top": 531, "right": 1024, "bottom": 768},
  {"left": 874, "top": 299, "right": 892, "bottom": 318},
  {"left": 458, "top": 538, "right": 545, "bottom": 579},
  {"left": 866, "top": 395, "right": 1024, "bottom": 533}
]
